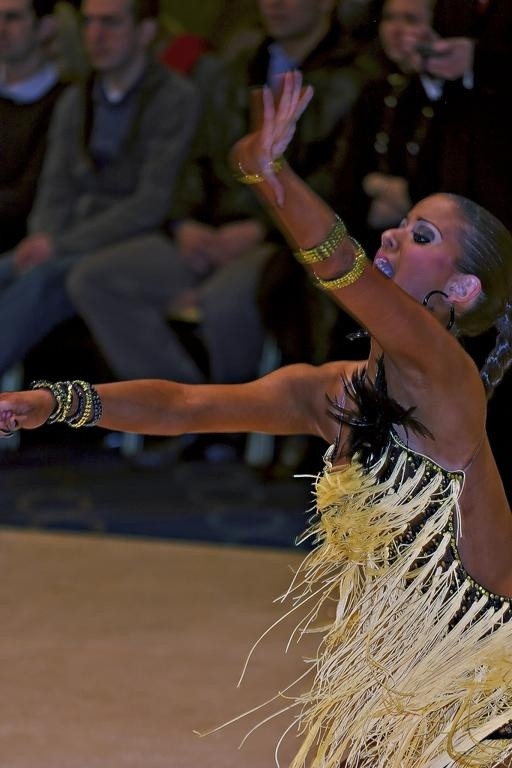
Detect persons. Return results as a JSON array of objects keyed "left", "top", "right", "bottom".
[
  {"left": 0, "top": 1, "right": 512, "bottom": 519},
  {"left": 0, "top": 64, "right": 512, "bottom": 768}
]
